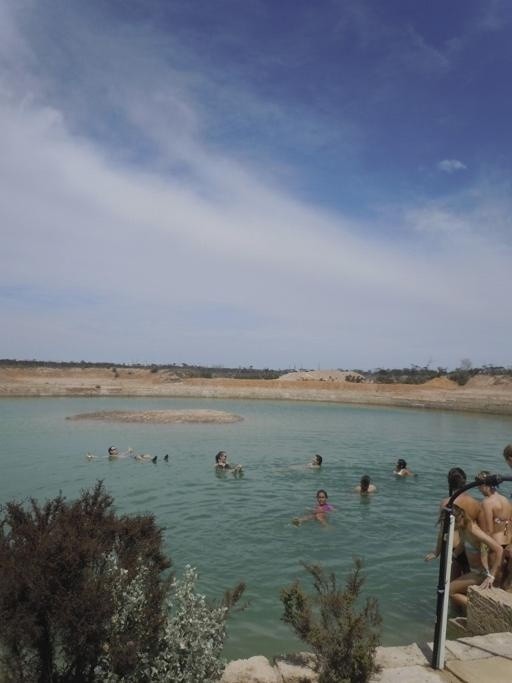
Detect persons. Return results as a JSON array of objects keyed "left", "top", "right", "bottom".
[
  {"left": 213, "top": 450, "right": 243, "bottom": 473},
  {"left": 475, "top": 470, "right": 511, "bottom": 564},
  {"left": 436, "top": 503, "right": 503, "bottom": 608},
  {"left": 303, "top": 453, "right": 322, "bottom": 469},
  {"left": 424, "top": 467, "right": 482, "bottom": 584},
  {"left": 502, "top": 442, "right": 512, "bottom": 468},
  {"left": 353, "top": 475, "right": 376, "bottom": 495},
  {"left": 87, "top": 445, "right": 168, "bottom": 463},
  {"left": 292, "top": 489, "right": 336, "bottom": 529},
  {"left": 391, "top": 458, "right": 415, "bottom": 477}
]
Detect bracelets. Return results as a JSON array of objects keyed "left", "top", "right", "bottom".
[{"left": 486, "top": 571, "right": 495, "bottom": 579}]
